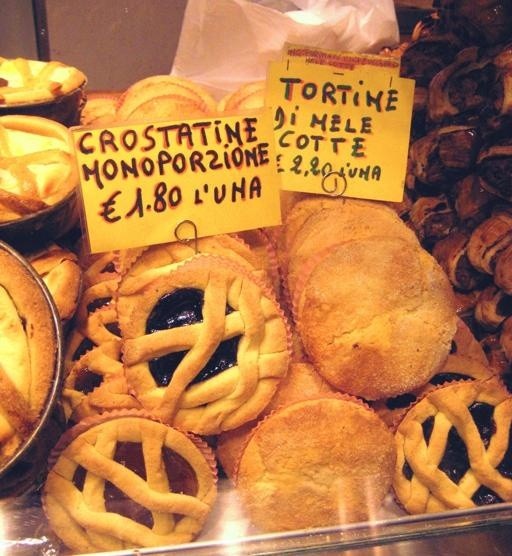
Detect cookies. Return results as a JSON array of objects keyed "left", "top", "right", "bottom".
[{"left": 39, "top": 75, "right": 511, "bottom": 552}]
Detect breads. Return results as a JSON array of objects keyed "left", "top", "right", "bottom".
[{"left": 381, "top": 0, "right": 511, "bottom": 392}]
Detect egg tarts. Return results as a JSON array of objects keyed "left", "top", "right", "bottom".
[
  {"left": 0, "top": 115, "right": 81, "bottom": 223},
  {"left": 0, "top": 53, "right": 84, "bottom": 103},
  {"left": 21, "top": 242, "right": 80, "bottom": 320},
  {"left": 0, "top": 250, "right": 58, "bottom": 470}
]
